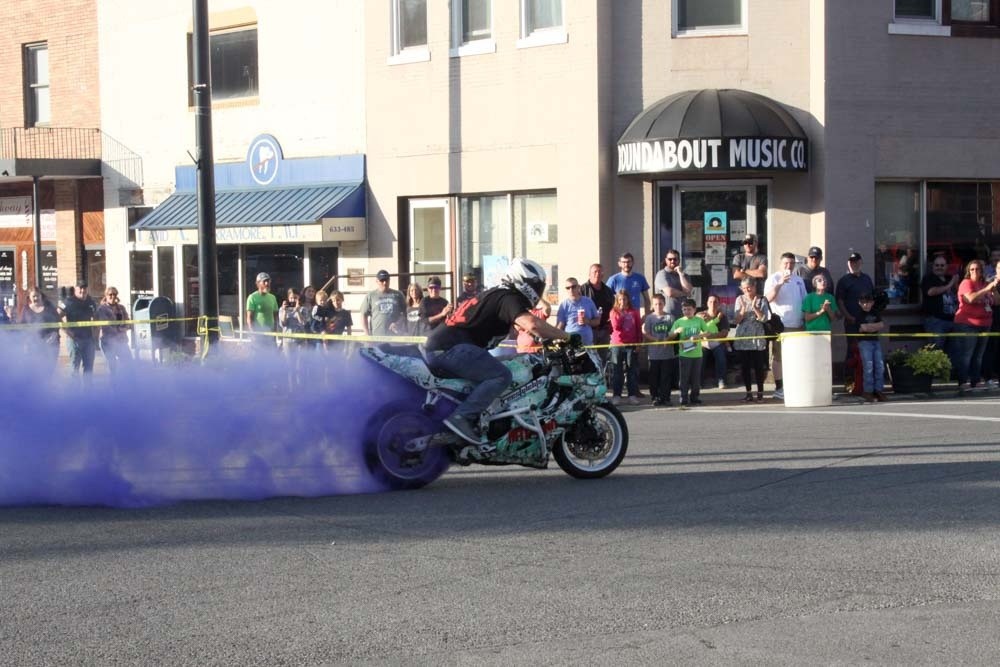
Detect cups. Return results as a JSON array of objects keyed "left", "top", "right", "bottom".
[{"left": 577, "top": 310, "right": 585, "bottom": 325}]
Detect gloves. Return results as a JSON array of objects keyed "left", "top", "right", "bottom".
[{"left": 562, "top": 333, "right": 583, "bottom": 347}]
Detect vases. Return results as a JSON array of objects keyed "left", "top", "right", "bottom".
[{"left": 890, "top": 366, "right": 932, "bottom": 393}]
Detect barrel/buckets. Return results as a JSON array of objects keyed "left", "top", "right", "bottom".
[{"left": 780, "top": 331, "right": 833, "bottom": 408}]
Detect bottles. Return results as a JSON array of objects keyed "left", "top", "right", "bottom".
[{"left": 597, "top": 306, "right": 603, "bottom": 319}]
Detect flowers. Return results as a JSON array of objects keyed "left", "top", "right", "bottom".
[{"left": 886, "top": 341, "right": 952, "bottom": 382}]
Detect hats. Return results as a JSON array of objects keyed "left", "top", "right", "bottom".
[
  {"left": 256, "top": 272, "right": 271, "bottom": 281},
  {"left": 75, "top": 280, "right": 89, "bottom": 288},
  {"left": 428, "top": 277, "right": 442, "bottom": 288},
  {"left": 848, "top": 253, "right": 862, "bottom": 261},
  {"left": 808, "top": 246, "right": 823, "bottom": 258},
  {"left": 376, "top": 270, "right": 391, "bottom": 280},
  {"left": 462, "top": 273, "right": 475, "bottom": 282}
]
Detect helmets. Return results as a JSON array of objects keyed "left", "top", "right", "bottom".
[{"left": 501, "top": 257, "right": 547, "bottom": 309}]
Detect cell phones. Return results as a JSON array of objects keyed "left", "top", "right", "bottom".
[{"left": 785, "top": 270, "right": 790, "bottom": 276}]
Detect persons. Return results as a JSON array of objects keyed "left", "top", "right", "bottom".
[
  {"left": 96, "top": 286, "right": 133, "bottom": 368},
  {"left": 642, "top": 250, "right": 729, "bottom": 407},
  {"left": 922, "top": 256, "right": 1000, "bottom": 392},
  {"left": 57, "top": 281, "right": 97, "bottom": 377},
  {"left": 515, "top": 299, "right": 551, "bottom": 352},
  {"left": 18, "top": 287, "right": 60, "bottom": 377},
  {"left": 553, "top": 251, "right": 650, "bottom": 404},
  {"left": 360, "top": 270, "right": 477, "bottom": 335},
  {"left": 421, "top": 259, "right": 583, "bottom": 444},
  {"left": 279, "top": 287, "right": 353, "bottom": 393},
  {"left": 247, "top": 272, "right": 280, "bottom": 350},
  {"left": 733, "top": 235, "right": 888, "bottom": 402}
]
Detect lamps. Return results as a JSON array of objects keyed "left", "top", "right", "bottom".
[
  {"left": 2, "top": 170, "right": 9, "bottom": 176},
  {"left": 1, "top": 252, "right": 7, "bottom": 257},
  {"left": 47, "top": 252, "right": 52, "bottom": 257},
  {"left": 94, "top": 250, "right": 102, "bottom": 256}
]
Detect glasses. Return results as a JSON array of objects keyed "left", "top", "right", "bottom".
[
  {"left": 934, "top": 264, "right": 947, "bottom": 267},
  {"left": 665, "top": 257, "right": 679, "bottom": 261},
  {"left": 743, "top": 240, "right": 756, "bottom": 245},
  {"left": 108, "top": 293, "right": 118, "bottom": 296},
  {"left": 565, "top": 285, "right": 579, "bottom": 290},
  {"left": 815, "top": 277, "right": 828, "bottom": 282}
]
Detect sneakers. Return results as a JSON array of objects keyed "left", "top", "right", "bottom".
[{"left": 442, "top": 409, "right": 482, "bottom": 445}]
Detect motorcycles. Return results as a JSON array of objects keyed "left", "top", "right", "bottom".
[{"left": 357, "top": 329, "right": 631, "bottom": 492}]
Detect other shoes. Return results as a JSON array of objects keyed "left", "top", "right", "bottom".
[
  {"left": 690, "top": 396, "right": 703, "bottom": 405},
  {"left": 875, "top": 391, "right": 888, "bottom": 402},
  {"left": 973, "top": 382, "right": 990, "bottom": 391},
  {"left": 741, "top": 396, "right": 754, "bottom": 403},
  {"left": 653, "top": 398, "right": 662, "bottom": 406},
  {"left": 679, "top": 397, "right": 687, "bottom": 404},
  {"left": 627, "top": 396, "right": 642, "bottom": 405},
  {"left": 863, "top": 393, "right": 877, "bottom": 402},
  {"left": 610, "top": 396, "right": 623, "bottom": 406},
  {"left": 959, "top": 383, "right": 972, "bottom": 393},
  {"left": 757, "top": 394, "right": 763, "bottom": 403},
  {"left": 664, "top": 398, "right": 674, "bottom": 406},
  {"left": 718, "top": 379, "right": 728, "bottom": 389}
]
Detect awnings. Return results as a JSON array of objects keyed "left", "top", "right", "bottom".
[
  {"left": 130, "top": 183, "right": 367, "bottom": 245},
  {"left": 616, "top": 88, "right": 807, "bottom": 178}
]
